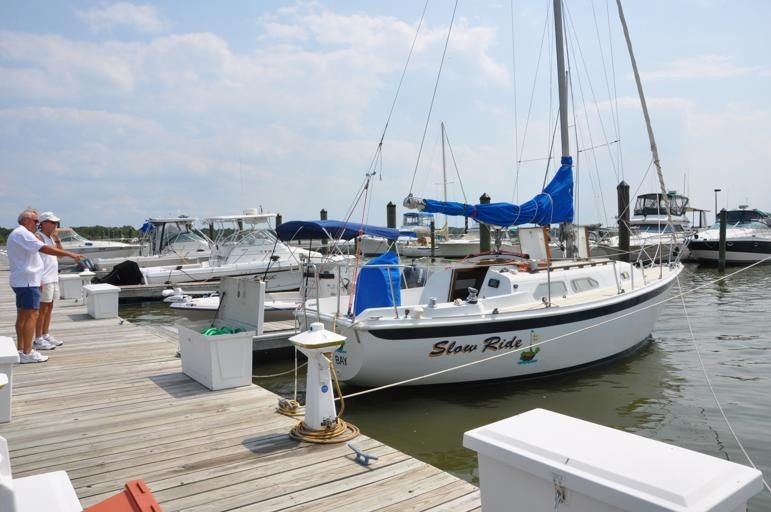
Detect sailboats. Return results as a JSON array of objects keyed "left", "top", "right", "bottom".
[{"left": 293, "top": 2, "right": 682, "bottom": 386}]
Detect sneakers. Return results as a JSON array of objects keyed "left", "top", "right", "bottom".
[
  {"left": 19, "top": 348, "right": 49, "bottom": 364},
  {"left": 32, "top": 337, "right": 57, "bottom": 350},
  {"left": 44, "top": 334, "right": 64, "bottom": 346}
]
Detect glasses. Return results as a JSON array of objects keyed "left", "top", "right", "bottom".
[
  {"left": 44, "top": 220, "right": 56, "bottom": 224},
  {"left": 31, "top": 218, "right": 38, "bottom": 224}
]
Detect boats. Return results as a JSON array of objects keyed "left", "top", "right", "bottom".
[
  {"left": 601, "top": 190, "right": 695, "bottom": 268},
  {"left": 162, "top": 254, "right": 360, "bottom": 322},
  {"left": 55, "top": 227, "right": 148, "bottom": 267},
  {"left": 55, "top": 213, "right": 401, "bottom": 302},
  {"left": 686, "top": 204, "right": 771, "bottom": 271}
]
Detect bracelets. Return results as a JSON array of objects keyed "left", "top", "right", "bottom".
[{"left": 55, "top": 239, "right": 60, "bottom": 243}]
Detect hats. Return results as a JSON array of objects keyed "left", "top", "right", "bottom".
[{"left": 39, "top": 211, "right": 61, "bottom": 223}]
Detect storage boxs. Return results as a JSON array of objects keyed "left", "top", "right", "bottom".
[
  {"left": 462, "top": 407, "right": 764, "bottom": 512},
  {"left": 174, "top": 275, "right": 266, "bottom": 391},
  {"left": 58, "top": 274, "right": 121, "bottom": 320}
]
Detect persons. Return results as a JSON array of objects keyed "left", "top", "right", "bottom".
[
  {"left": 7, "top": 206, "right": 88, "bottom": 364},
  {"left": 32, "top": 212, "right": 63, "bottom": 350}
]
